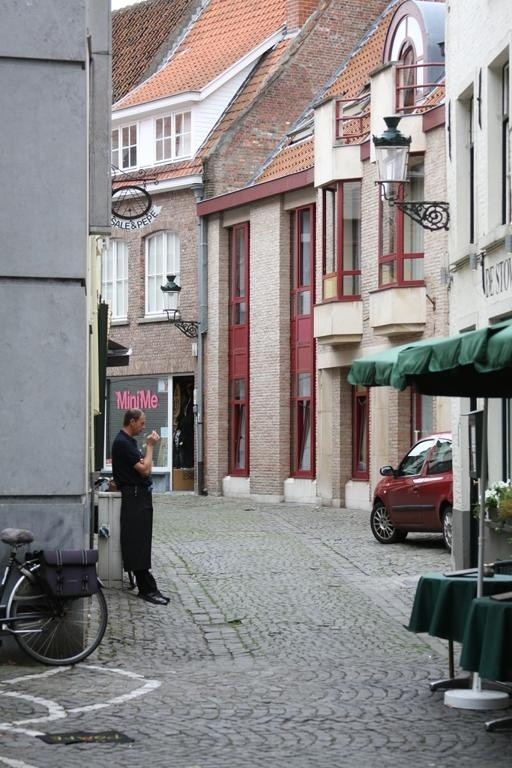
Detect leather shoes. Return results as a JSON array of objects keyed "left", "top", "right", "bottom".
[{"left": 138, "top": 589, "right": 169, "bottom": 604}]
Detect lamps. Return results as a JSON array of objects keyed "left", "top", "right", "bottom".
[
  {"left": 160, "top": 275, "right": 198, "bottom": 338},
  {"left": 372, "top": 116, "right": 449, "bottom": 231}
]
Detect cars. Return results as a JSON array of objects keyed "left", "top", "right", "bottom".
[{"left": 370, "top": 435, "right": 453, "bottom": 551}]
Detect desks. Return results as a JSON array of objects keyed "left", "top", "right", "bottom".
[
  {"left": 404, "top": 573, "right": 512, "bottom": 693},
  {"left": 460, "top": 594, "right": 512, "bottom": 733}
]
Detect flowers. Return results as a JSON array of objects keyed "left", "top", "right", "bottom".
[{"left": 471, "top": 477, "right": 512, "bottom": 526}]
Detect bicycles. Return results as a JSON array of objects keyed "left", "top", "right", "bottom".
[{"left": 0, "top": 529, "right": 108, "bottom": 664}]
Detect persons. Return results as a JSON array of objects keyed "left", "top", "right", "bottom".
[{"left": 110, "top": 405, "right": 172, "bottom": 608}]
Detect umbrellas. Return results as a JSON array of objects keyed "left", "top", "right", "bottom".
[
  {"left": 388, "top": 316, "right": 512, "bottom": 392},
  {"left": 344, "top": 330, "right": 491, "bottom": 600}
]
